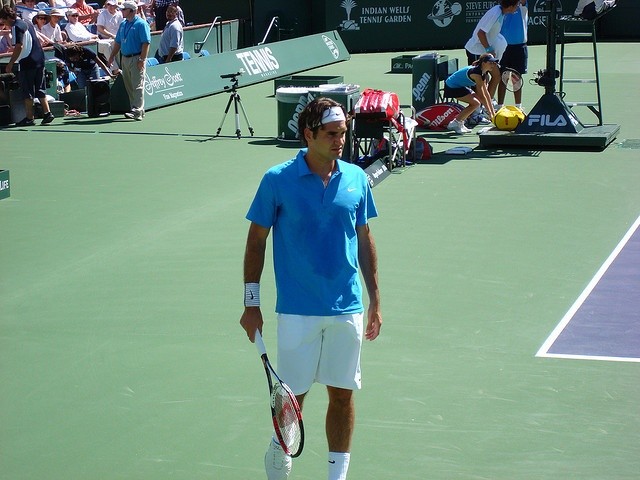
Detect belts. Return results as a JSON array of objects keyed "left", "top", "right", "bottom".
[{"left": 124, "top": 52, "right": 142, "bottom": 58}]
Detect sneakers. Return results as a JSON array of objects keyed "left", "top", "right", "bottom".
[
  {"left": 470, "top": 113, "right": 491, "bottom": 125},
  {"left": 40, "top": 111, "right": 54, "bottom": 125},
  {"left": 462, "top": 122, "right": 471, "bottom": 133},
  {"left": 135, "top": 111, "right": 144, "bottom": 120},
  {"left": 15, "top": 116, "right": 36, "bottom": 126},
  {"left": 125, "top": 111, "right": 135, "bottom": 118},
  {"left": 446, "top": 121, "right": 467, "bottom": 135},
  {"left": 265, "top": 445, "right": 292, "bottom": 476}
]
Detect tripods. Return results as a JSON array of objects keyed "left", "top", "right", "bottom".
[{"left": 214, "top": 71, "right": 255, "bottom": 139}]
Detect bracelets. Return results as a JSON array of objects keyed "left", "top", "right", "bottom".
[
  {"left": 484, "top": 46, "right": 494, "bottom": 53},
  {"left": 138, "top": 57, "right": 146, "bottom": 63},
  {"left": 244, "top": 283, "right": 261, "bottom": 308}
]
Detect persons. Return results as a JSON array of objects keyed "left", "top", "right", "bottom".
[
  {"left": 64, "top": 8, "right": 122, "bottom": 76},
  {"left": 0, "top": 19, "right": 14, "bottom": 53},
  {"left": 29, "top": 11, "right": 54, "bottom": 47},
  {"left": 41, "top": 9, "right": 66, "bottom": 45},
  {"left": 107, "top": 1, "right": 151, "bottom": 121},
  {"left": 44, "top": 58, "right": 71, "bottom": 94},
  {"left": 465, "top": 0, "right": 518, "bottom": 125},
  {"left": 113, "top": 67, "right": 122, "bottom": 77},
  {"left": 241, "top": 97, "right": 383, "bottom": 480},
  {"left": 4, "top": 4, "right": 21, "bottom": 20},
  {"left": 158, "top": 6, "right": 184, "bottom": 63},
  {"left": 61, "top": 44, "right": 118, "bottom": 89},
  {"left": 497, "top": 0, "right": 528, "bottom": 117},
  {"left": 150, "top": 0, "right": 179, "bottom": 31},
  {"left": 96, "top": 1, "right": 124, "bottom": 40},
  {"left": 443, "top": 53, "right": 500, "bottom": 134},
  {"left": 70, "top": 0, "right": 101, "bottom": 26},
  {"left": 15, "top": 0, "right": 45, "bottom": 28},
  {"left": 0, "top": 5, "right": 54, "bottom": 126}
]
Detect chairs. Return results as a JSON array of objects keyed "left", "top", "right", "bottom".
[
  {"left": 438, "top": 58, "right": 459, "bottom": 103},
  {"left": 351, "top": 95, "right": 406, "bottom": 173},
  {"left": 365, "top": 104, "right": 416, "bottom": 165},
  {"left": 1, "top": 0, "right": 212, "bottom": 92}
]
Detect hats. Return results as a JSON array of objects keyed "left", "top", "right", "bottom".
[
  {"left": 105, "top": 1, "right": 118, "bottom": 5},
  {"left": 32, "top": 11, "right": 51, "bottom": 25},
  {"left": 49, "top": 9, "right": 65, "bottom": 18},
  {"left": 117, "top": 2, "right": 138, "bottom": 11},
  {"left": 479, "top": 54, "right": 499, "bottom": 63},
  {"left": 67, "top": 9, "right": 79, "bottom": 15}
]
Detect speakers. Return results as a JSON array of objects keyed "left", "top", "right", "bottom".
[{"left": 86, "top": 79, "right": 111, "bottom": 117}]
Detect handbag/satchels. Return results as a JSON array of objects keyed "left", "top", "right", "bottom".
[
  {"left": 408, "top": 137, "right": 432, "bottom": 160},
  {"left": 350, "top": 88, "right": 399, "bottom": 120},
  {"left": 411, "top": 103, "right": 469, "bottom": 130},
  {"left": 492, "top": 105, "right": 527, "bottom": 129}
]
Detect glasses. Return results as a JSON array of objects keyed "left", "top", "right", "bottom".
[{"left": 36, "top": 17, "right": 48, "bottom": 20}]
